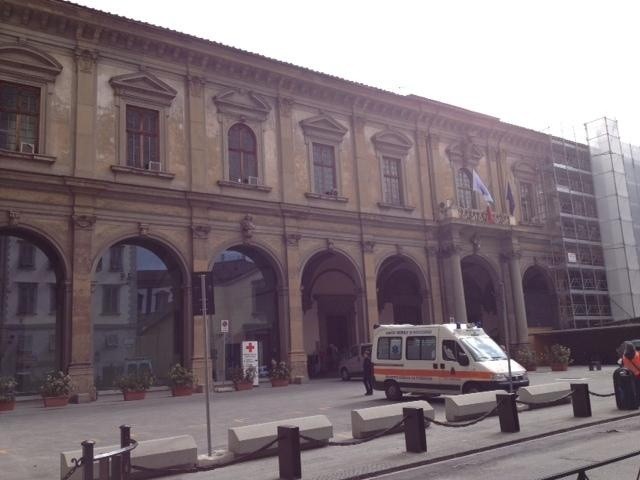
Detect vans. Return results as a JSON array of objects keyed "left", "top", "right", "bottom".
[{"left": 338, "top": 342, "right": 374, "bottom": 380}]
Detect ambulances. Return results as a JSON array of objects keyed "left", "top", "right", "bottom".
[
  {"left": 123, "top": 356, "right": 156, "bottom": 381},
  {"left": 368, "top": 321, "right": 530, "bottom": 403}
]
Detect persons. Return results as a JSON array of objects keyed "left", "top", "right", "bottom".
[
  {"left": 362, "top": 350, "right": 373, "bottom": 395},
  {"left": 622, "top": 343, "right": 640, "bottom": 383}
]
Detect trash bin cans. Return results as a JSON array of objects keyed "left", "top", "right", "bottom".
[
  {"left": 613, "top": 368, "right": 639, "bottom": 411},
  {"left": 308, "top": 355, "right": 320, "bottom": 379}
]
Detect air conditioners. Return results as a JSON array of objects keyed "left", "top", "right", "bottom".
[{"left": 438, "top": 200, "right": 453, "bottom": 211}]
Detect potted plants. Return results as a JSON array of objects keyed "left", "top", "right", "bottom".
[
  {"left": 168, "top": 363, "right": 194, "bottom": 397},
  {"left": 118, "top": 370, "right": 156, "bottom": 400},
  {"left": 551, "top": 343, "right": 571, "bottom": 371},
  {"left": 39, "top": 371, "right": 71, "bottom": 407},
  {"left": 517, "top": 350, "right": 546, "bottom": 371},
  {"left": 265, "top": 359, "right": 290, "bottom": 387},
  {"left": 0, "top": 375, "right": 18, "bottom": 411},
  {"left": 229, "top": 364, "right": 257, "bottom": 390}
]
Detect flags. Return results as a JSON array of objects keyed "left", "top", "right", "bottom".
[
  {"left": 487, "top": 206, "right": 495, "bottom": 224},
  {"left": 506, "top": 182, "right": 515, "bottom": 215},
  {"left": 472, "top": 169, "right": 493, "bottom": 203}
]
